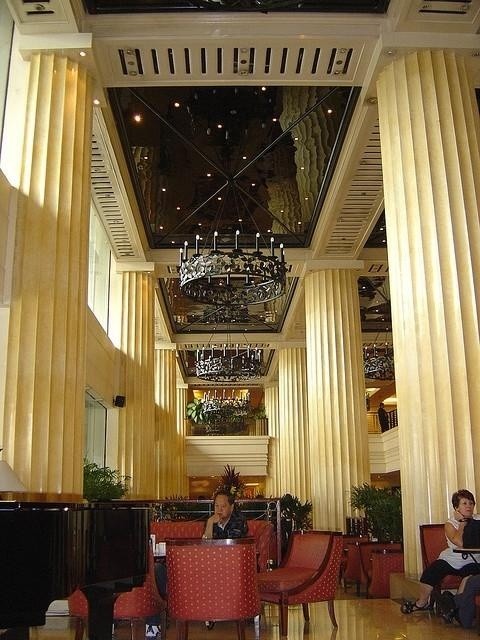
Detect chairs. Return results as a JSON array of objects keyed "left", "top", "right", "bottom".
[
  {"left": 420, "top": 525, "right": 463, "bottom": 624},
  {"left": 70, "top": 540, "right": 166, "bottom": 639},
  {"left": 342, "top": 534, "right": 404, "bottom": 600},
  {"left": 473, "top": 595, "right": 480, "bottom": 636},
  {"left": 167, "top": 544, "right": 265, "bottom": 639},
  {"left": 152, "top": 520, "right": 275, "bottom": 572},
  {"left": 258, "top": 530, "right": 345, "bottom": 637}
]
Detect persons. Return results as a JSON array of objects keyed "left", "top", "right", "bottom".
[
  {"left": 438, "top": 569, "right": 480, "bottom": 630},
  {"left": 199, "top": 489, "right": 250, "bottom": 543},
  {"left": 398, "top": 485, "right": 480, "bottom": 616},
  {"left": 141, "top": 558, "right": 168, "bottom": 640}
]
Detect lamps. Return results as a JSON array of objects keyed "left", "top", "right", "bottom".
[
  {"left": 176, "top": 180, "right": 293, "bottom": 423},
  {"left": 0, "top": 461, "right": 27, "bottom": 491},
  {"left": 186, "top": 86, "right": 276, "bottom": 178},
  {"left": 363, "top": 332, "right": 395, "bottom": 380}
]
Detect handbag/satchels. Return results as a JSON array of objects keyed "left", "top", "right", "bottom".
[{"left": 461, "top": 518, "right": 480, "bottom": 549}]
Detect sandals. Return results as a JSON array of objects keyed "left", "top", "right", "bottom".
[{"left": 401, "top": 598, "right": 454, "bottom": 623}]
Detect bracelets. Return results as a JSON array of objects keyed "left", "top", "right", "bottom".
[{"left": 202, "top": 533, "right": 210, "bottom": 540}]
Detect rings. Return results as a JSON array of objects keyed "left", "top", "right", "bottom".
[{"left": 457, "top": 512, "right": 460, "bottom": 514}]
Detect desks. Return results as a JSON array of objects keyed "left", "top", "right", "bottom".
[{"left": 453, "top": 549, "right": 480, "bottom": 563}]
